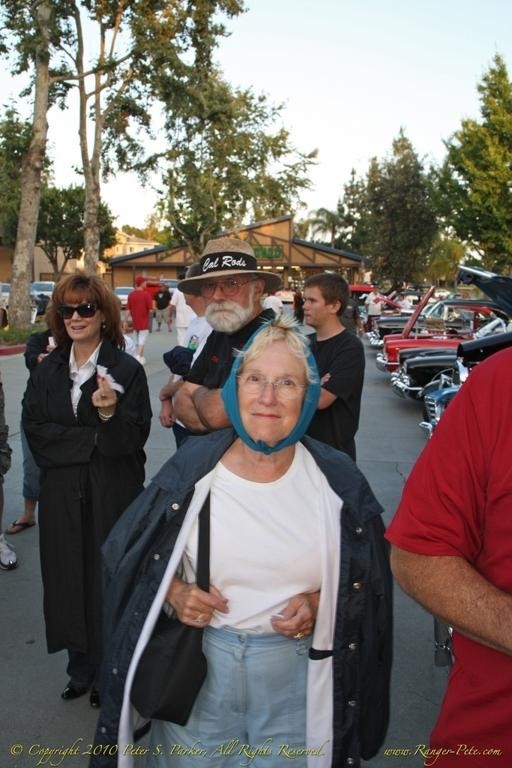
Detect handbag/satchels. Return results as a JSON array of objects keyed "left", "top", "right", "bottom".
[{"left": 129, "top": 613, "right": 206, "bottom": 726}]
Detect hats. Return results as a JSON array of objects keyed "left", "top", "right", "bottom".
[
  {"left": 177, "top": 238, "right": 281, "bottom": 296},
  {"left": 136, "top": 276, "right": 148, "bottom": 284}
]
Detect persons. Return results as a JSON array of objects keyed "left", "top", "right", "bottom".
[{"left": 0, "top": 236, "right": 512, "bottom": 768}]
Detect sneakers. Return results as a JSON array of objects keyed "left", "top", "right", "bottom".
[
  {"left": 135, "top": 356, "right": 145, "bottom": 365},
  {"left": 0, "top": 533, "right": 17, "bottom": 570}
]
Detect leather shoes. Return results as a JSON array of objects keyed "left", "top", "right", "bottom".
[{"left": 61, "top": 680, "right": 101, "bottom": 708}]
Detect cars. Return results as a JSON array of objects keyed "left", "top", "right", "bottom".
[
  {"left": 0, "top": 274, "right": 181, "bottom": 326},
  {"left": 349, "top": 262, "right": 510, "bottom": 437}
]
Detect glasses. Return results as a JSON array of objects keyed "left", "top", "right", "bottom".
[
  {"left": 200, "top": 277, "right": 260, "bottom": 298},
  {"left": 237, "top": 374, "right": 304, "bottom": 397},
  {"left": 58, "top": 304, "right": 99, "bottom": 319}
]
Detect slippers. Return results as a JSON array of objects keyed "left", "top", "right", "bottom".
[{"left": 5, "top": 519, "right": 35, "bottom": 534}]
